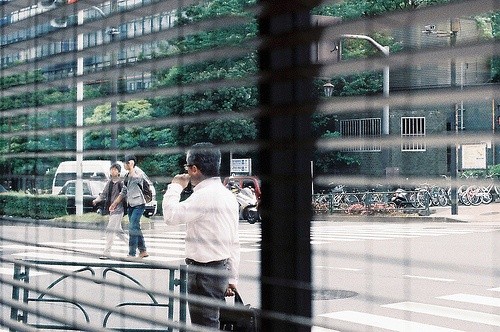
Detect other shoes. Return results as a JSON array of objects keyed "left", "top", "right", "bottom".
[
  {"left": 99, "top": 256, "right": 112, "bottom": 259},
  {"left": 137, "top": 251, "right": 149, "bottom": 259}
]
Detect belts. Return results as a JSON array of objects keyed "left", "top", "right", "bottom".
[{"left": 185, "top": 258, "right": 228, "bottom": 267}]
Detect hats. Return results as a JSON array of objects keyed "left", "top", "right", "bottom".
[{"left": 120, "top": 153, "right": 138, "bottom": 163}]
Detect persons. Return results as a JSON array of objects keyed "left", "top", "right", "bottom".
[
  {"left": 109, "top": 154, "right": 149, "bottom": 261},
  {"left": 92, "top": 163, "right": 130, "bottom": 260},
  {"left": 161, "top": 142, "right": 239, "bottom": 328}
]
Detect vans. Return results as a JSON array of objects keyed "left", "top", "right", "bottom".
[{"left": 52, "top": 160, "right": 158, "bottom": 216}]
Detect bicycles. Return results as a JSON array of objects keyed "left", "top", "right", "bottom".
[{"left": 312, "top": 170, "right": 500, "bottom": 216}]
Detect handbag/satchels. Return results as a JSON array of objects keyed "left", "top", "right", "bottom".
[
  {"left": 218, "top": 287, "right": 258, "bottom": 332},
  {"left": 136, "top": 178, "right": 153, "bottom": 204}
]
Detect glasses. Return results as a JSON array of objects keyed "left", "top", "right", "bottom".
[{"left": 183, "top": 163, "right": 196, "bottom": 170}]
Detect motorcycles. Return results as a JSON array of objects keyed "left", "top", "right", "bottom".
[{"left": 225, "top": 187, "right": 258, "bottom": 224}]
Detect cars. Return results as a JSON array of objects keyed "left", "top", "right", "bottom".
[
  {"left": 57, "top": 180, "right": 155, "bottom": 219},
  {"left": 223, "top": 174, "right": 262, "bottom": 218}
]
